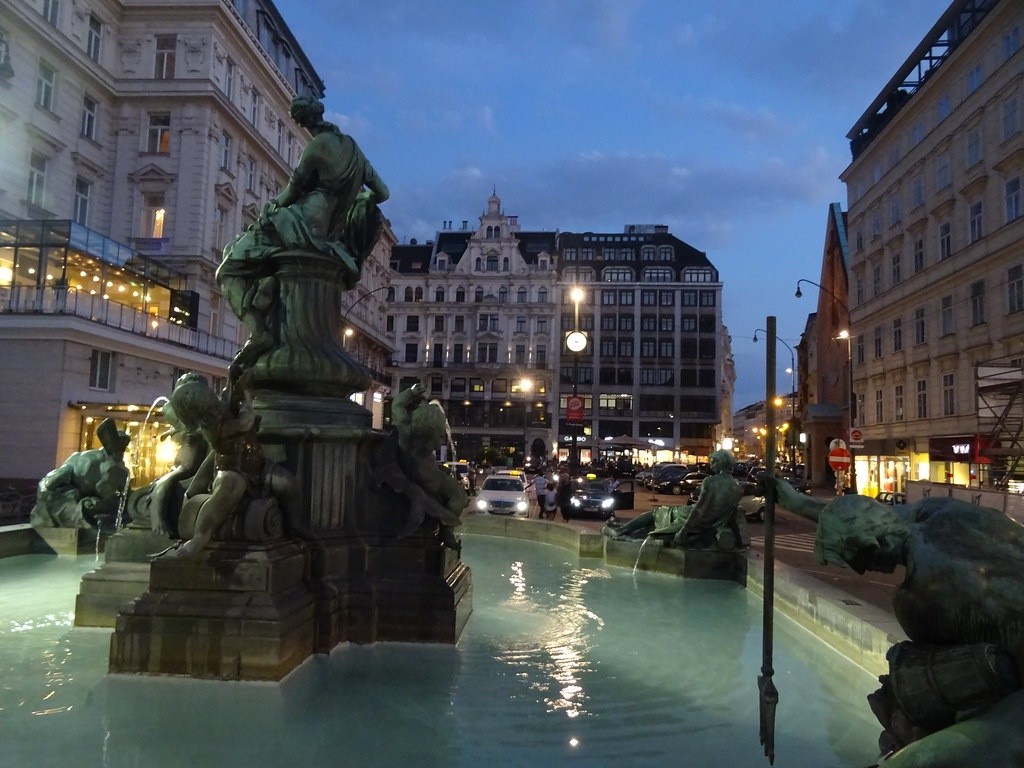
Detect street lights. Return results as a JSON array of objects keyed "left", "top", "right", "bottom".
[
  {"left": 752, "top": 328, "right": 796, "bottom": 474},
  {"left": 566, "top": 284, "right": 586, "bottom": 475},
  {"left": 519, "top": 380, "right": 531, "bottom": 453},
  {"left": 796, "top": 278, "right": 853, "bottom": 427}
]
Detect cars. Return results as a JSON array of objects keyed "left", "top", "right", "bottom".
[
  {"left": 444, "top": 462, "right": 473, "bottom": 492},
  {"left": 566, "top": 476, "right": 635, "bottom": 520},
  {"left": 733, "top": 458, "right": 806, "bottom": 521},
  {"left": 636, "top": 462, "right": 708, "bottom": 495},
  {"left": 474, "top": 469, "right": 530, "bottom": 518}
]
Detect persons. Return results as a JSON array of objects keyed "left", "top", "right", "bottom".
[
  {"left": 599, "top": 449, "right": 747, "bottom": 547},
  {"left": 607, "top": 474, "right": 622, "bottom": 517},
  {"left": 391, "top": 382, "right": 471, "bottom": 515},
  {"left": 753, "top": 471, "right": 1024, "bottom": 756},
  {"left": 147, "top": 371, "right": 316, "bottom": 561},
  {"left": 524, "top": 470, "right": 574, "bottom": 522},
  {"left": 215, "top": 94, "right": 389, "bottom": 364}
]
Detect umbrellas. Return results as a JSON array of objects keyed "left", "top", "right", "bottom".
[{"left": 565, "top": 433, "right": 652, "bottom": 465}]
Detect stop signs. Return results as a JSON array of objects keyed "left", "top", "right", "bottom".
[{"left": 828, "top": 448, "right": 853, "bottom": 471}]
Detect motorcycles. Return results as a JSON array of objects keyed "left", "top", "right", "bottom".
[{"left": 545, "top": 481, "right": 558, "bottom": 521}]
